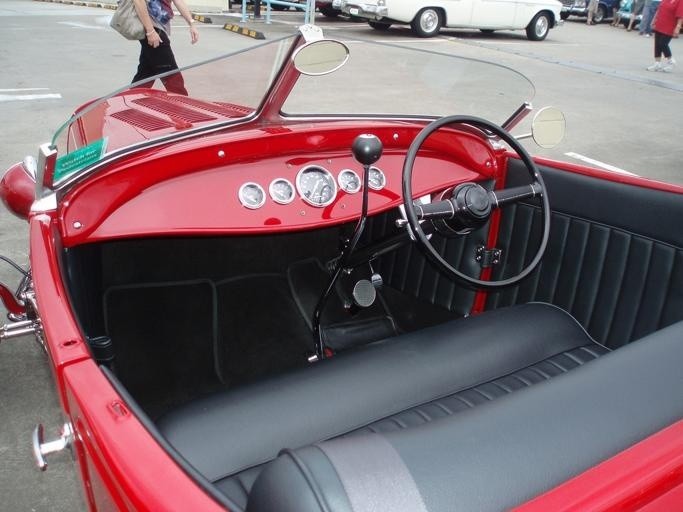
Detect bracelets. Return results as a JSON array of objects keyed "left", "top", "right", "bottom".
[
  {"left": 189, "top": 19, "right": 194, "bottom": 24},
  {"left": 146, "top": 30, "right": 156, "bottom": 35}
]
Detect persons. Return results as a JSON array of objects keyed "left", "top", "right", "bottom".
[
  {"left": 645, "top": 0, "right": 683, "bottom": 72},
  {"left": 587, "top": 0, "right": 660, "bottom": 38},
  {"left": 110, "top": 0, "right": 200, "bottom": 96}
]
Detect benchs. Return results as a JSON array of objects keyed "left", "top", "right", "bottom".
[{"left": 156, "top": 298, "right": 683, "bottom": 511}]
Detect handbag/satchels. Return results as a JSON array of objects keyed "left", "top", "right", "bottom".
[{"left": 109, "top": 0, "right": 147, "bottom": 40}]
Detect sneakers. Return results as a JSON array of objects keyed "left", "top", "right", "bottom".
[
  {"left": 647, "top": 63, "right": 663, "bottom": 72},
  {"left": 663, "top": 59, "right": 674, "bottom": 71}
]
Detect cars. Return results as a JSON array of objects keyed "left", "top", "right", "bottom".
[
  {"left": 561, "top": 0, "right": 645, "bottom": 30},
  {"left": 330, "top": 0, "right": 567, "bottom": 41},
  {"left": 233, "top": 0, "right": 342, "bottom": 18}
]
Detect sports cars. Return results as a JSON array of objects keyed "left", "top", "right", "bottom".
[{"left": 0, "top": 25, "right": 683, "bottom": 512}]
